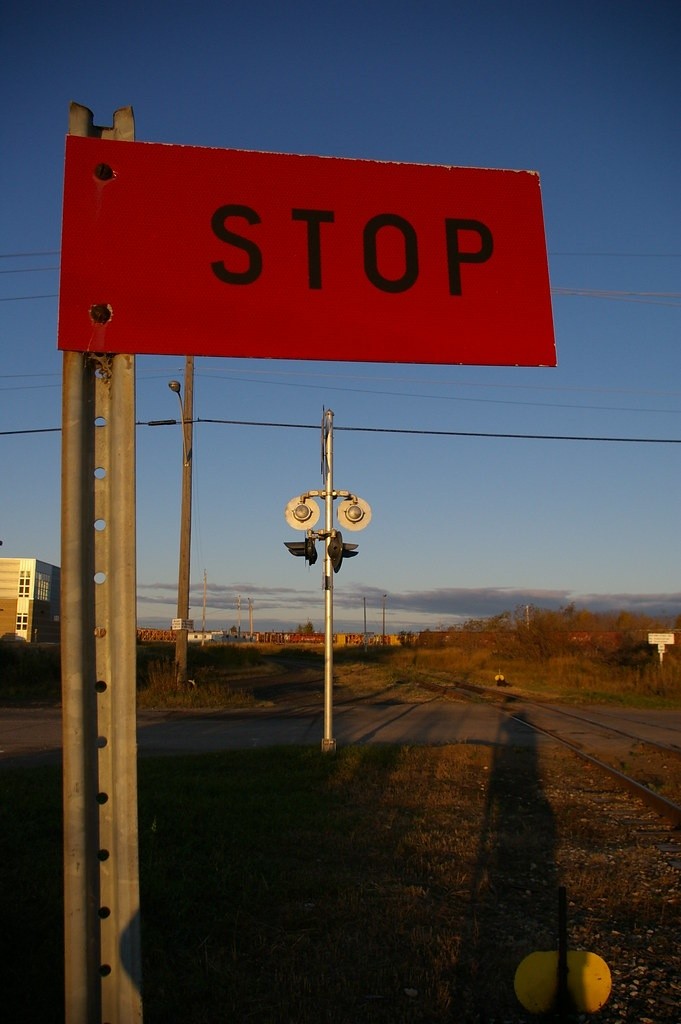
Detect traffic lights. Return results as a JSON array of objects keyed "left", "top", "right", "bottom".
[
  {"left": 283, "top": 530, "right": 319, "bottom": 567},
  {"left": 331, "top": 530, "right": 360, "bottom": 572}
]
